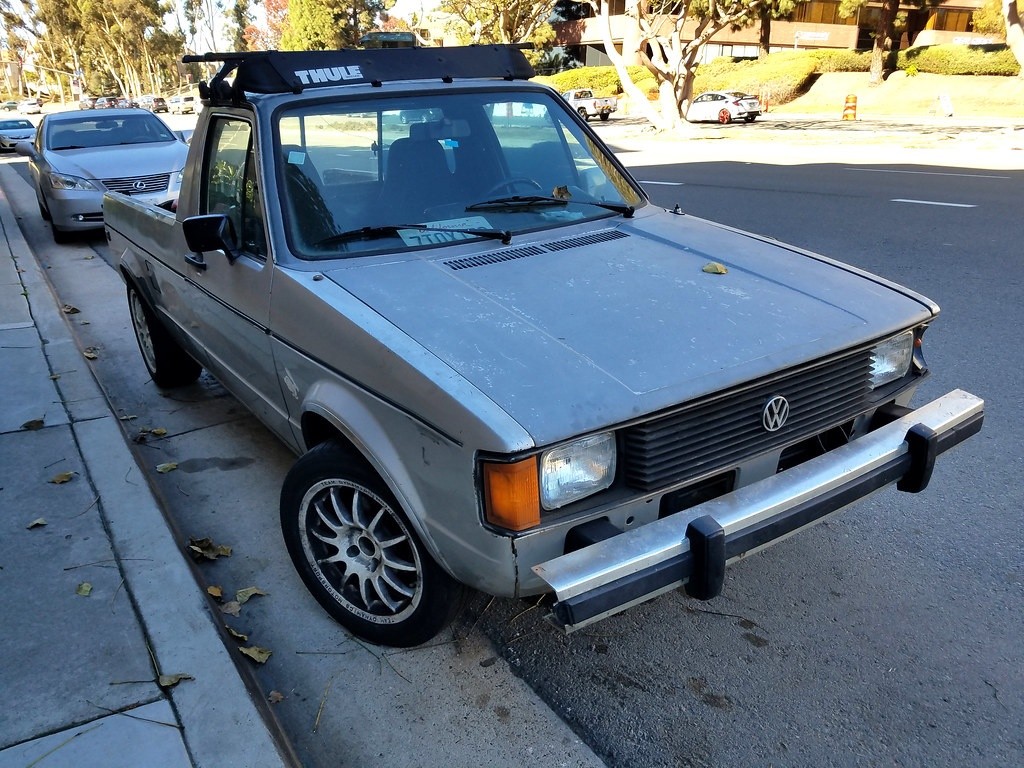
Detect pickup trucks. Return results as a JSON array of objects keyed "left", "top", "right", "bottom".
[
  {"left": 552, "top": 88, "right": 617, "bottom": 121},
  {"left": 102, "top": 41, "right": 986, "bottom": 649}
]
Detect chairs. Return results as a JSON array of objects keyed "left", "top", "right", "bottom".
[{"left": 376, "top": 138, "right": 454, "bottom": 215}]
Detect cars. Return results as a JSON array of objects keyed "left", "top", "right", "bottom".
[
  {"left": 0, "top": 119, "right": 37, "bottom": 152},
  {"left": 0, "top": 97, "right": 44, "bottom": 115},
  {"left": 78, "top": 95, "right": 203, "bottom": 115},
  {"left": 685, "top": 91, "right": 763, "bottom": 124},
  {"left": 15, "top": 109, "right": 191, "bottom": 245}
]
